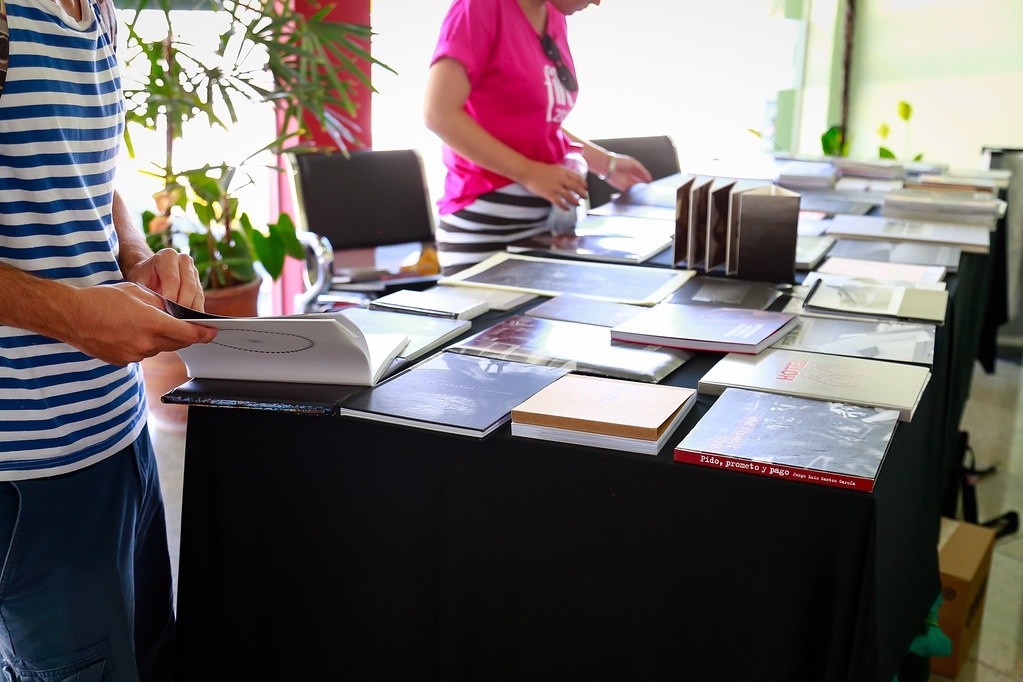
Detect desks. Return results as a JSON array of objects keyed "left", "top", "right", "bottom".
[{"left": 161, "top": 151, "right": 1010, "bottom": 682}]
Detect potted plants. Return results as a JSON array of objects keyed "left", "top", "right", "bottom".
[{"left": 120, "top": 1, "right": 399, "bottom": 436}]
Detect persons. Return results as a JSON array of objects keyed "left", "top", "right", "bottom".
[
  {"left": 1, "top": 1, "right": 220, "bottom": 682},
  {"left": 428, "top": 0, "right": 653, "bottom": 284}
]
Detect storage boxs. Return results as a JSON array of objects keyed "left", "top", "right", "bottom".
[{"left": 930, "top": 517, "right": 997, "bottom": 681}]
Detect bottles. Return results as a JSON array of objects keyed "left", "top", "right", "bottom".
[{"left": 550, "top": 158, "right": 581, "bottom": 238}]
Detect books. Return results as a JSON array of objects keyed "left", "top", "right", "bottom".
[
  {"left": 136, "top": 280, "right": 412, "bottom": 385},
  {"left": 674, "top": 387, "right": 900, "bottom": 494},
  {"left": 610, "top": 303, "right": 799, "bottom": 355},
  {"left": 341, "top": 351, "right": 573, "bottom": 440},
  {"left": 369, "top": 289, "right": 490, "bottom": 321},
  {"left": 160, "top": 357, "right": 411, "bottom": 418},
  {"left": 803, "top": 279, "right": 949, "bottom": 326},
  {"left": 330, "top": 307, "right": 472, "bottom": 360},
  {"left": 511, "top": 373, "right": 697, "bottom": 457},
  {"left": 781, "top": 271, "right": 949, "bottom": 327},
  {"left": 696, "top": 345, "right": 932, "bottom": 425},
  {"left": 418, "top": 284, "right": 542, "bottom": 310},
  {"left": 435, "top": 153, "right": 1011, "bottom": 305},
  {"left": 439, "top": 314, "right": 697, "bottom": 383},
  {"left": 764, "top": 310, "right": 936, "bottom": 371},
  {"left": 524, "top": 292, "right": 653, "bottom": 329}
]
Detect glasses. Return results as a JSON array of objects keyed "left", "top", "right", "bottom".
[{"left": 539, "top": 32, "right": 577, "bottom": 90}]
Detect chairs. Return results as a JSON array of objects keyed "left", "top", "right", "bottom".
[
  {"left": 281, "top": 148, "right": 444, "bottom": 315},
  {"left": 584, "top": 135, "right": 682, "bottom": 215}
]
